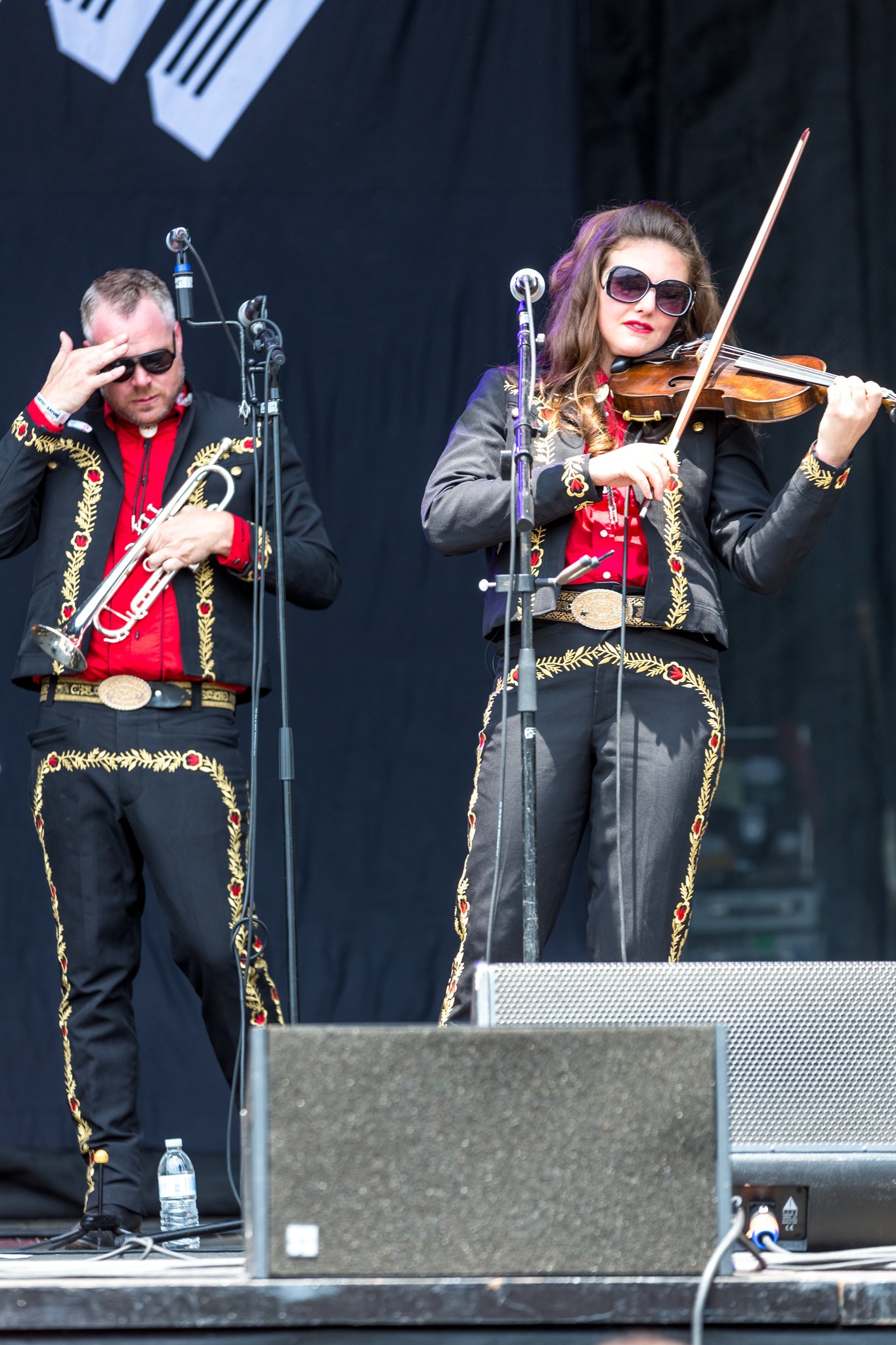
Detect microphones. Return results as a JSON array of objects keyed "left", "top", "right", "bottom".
[
  {"left": 237, "top": 295, "right": 268, "bottom": 327},
  {"left": 165, "top": 226, "right": 190, "bottom": 252},
  {"left": 510, "top": 268, "right": 545, "bottom": 304}
]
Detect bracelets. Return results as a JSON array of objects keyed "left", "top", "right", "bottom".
[{"left": 35, "top": 392, "right": 70, "bottom": 425}]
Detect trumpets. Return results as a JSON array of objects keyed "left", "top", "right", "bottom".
[{"left": 29, "top": 430, "right": 235, "bottom": 669}]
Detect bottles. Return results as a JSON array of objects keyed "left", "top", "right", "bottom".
[{"left": 157, "top": 1137, "right": 201, "bottom": 1250}]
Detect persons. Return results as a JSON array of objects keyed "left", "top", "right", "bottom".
[
  {"left": 422, "top": 201, "right": 883, "bottom": 1027},
  {"left": 0, "top": 268, "right": 342, "bottom": 1249}
]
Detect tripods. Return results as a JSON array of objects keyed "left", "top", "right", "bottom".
[
  {"left": 10, "top": 1146, "right": 181, "bottom": 1258},
  {"left": 113, "top": 366, "right": 357, "bottom": 1248}
]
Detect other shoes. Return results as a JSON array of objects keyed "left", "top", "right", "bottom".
[{"left": 65, "top": 1225, "right": 142, "bottom": 1250}]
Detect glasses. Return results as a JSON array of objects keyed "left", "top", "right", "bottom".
[
  {"left": 100, "top": 327, "right": 177, "bottom": 383},
  {"left": 602, "top": 265, "right": 698, "bottom": 317}
]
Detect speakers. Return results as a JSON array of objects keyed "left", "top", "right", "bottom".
[
  {"left": 247, "top": 1024, "right": 728, "bottom": 1275},
  {"left": 468, "top": 955, "right": 896, "bottom": 1244}
]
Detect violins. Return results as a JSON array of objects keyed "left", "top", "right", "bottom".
[{"left": 609, "top": 349, "right": 896, "bottom": 420}]
